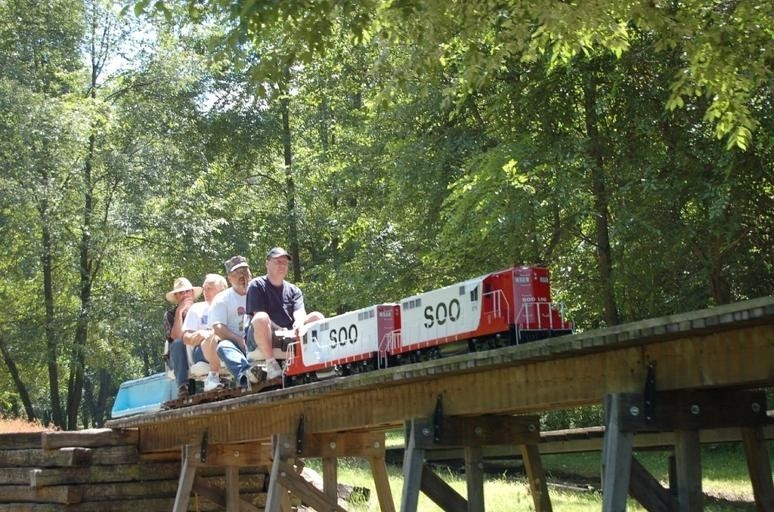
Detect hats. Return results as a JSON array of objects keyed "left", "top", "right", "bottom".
[
  {"left": 266, "top": 246, "right": 293, "bottom": 262},
  {"left": 224, "top": 255, "right": 250, "bottom": 275},
  {"left": 165, "top": 277, "right": 203, "bottom": 305}
]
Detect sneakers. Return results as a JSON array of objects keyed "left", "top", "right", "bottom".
[
  {"left": 265, "top": 358, "right": 284, "bottom": 382},
  {"left": 238, "top": 373, "right": 248, "bottom": 385},
  {"left": 248, "top": 365, "right": 264, "bottom": 384},
  {"left": 203, "top": 370, "right": 225, "bottom": 392},
  {"left": 177, "top": 385, "right": 190, "bottom": 397}
]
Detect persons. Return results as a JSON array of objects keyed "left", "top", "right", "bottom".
[
  {"left": 245, "top": 247, "right": 325, "bottom": 381},
  {"left": 208, "top": 255, "right": 267, "bottom": 384},
  {"left": 182, "top": 273, "right": 229, "bottom": 393},
  {"left": 160, "top": 277, "right": 202, "bottom": 398}
]
